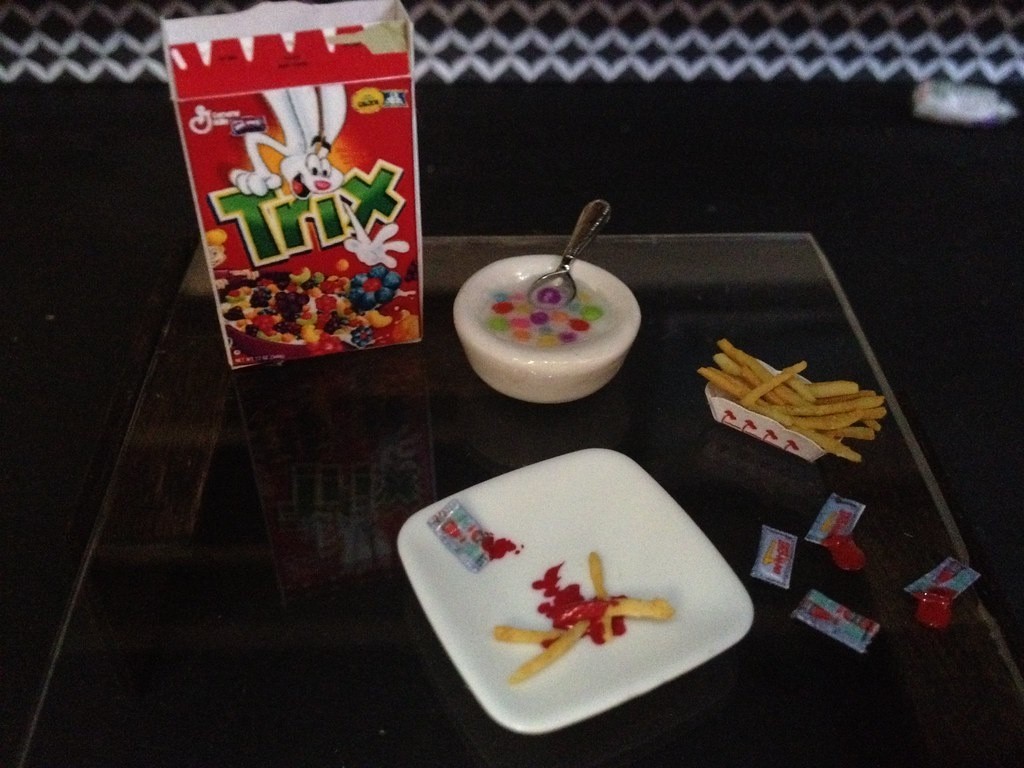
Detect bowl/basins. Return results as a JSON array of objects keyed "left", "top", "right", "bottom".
[{"left": 455, "top": 253, "right": 644, "bottom": 404}]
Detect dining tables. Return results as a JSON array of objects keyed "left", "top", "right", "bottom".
[{"left": 18, "top": 232, "right": 1023, "bottom": 768}]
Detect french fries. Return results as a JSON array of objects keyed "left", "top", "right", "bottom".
[
  {"left": 697, "top": 338, "right": 886, "bottom": 461},
  {"left": 493, "top": 554, "right": 671, "bottom": 681}
]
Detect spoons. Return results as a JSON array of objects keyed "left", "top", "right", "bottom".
[{"left": 527, "top": 199, "right": 611, "bottom": 309}]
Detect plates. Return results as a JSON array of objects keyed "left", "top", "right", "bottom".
[{"left": 396, "top": 448, "right": 756, "bottom": 737}]
